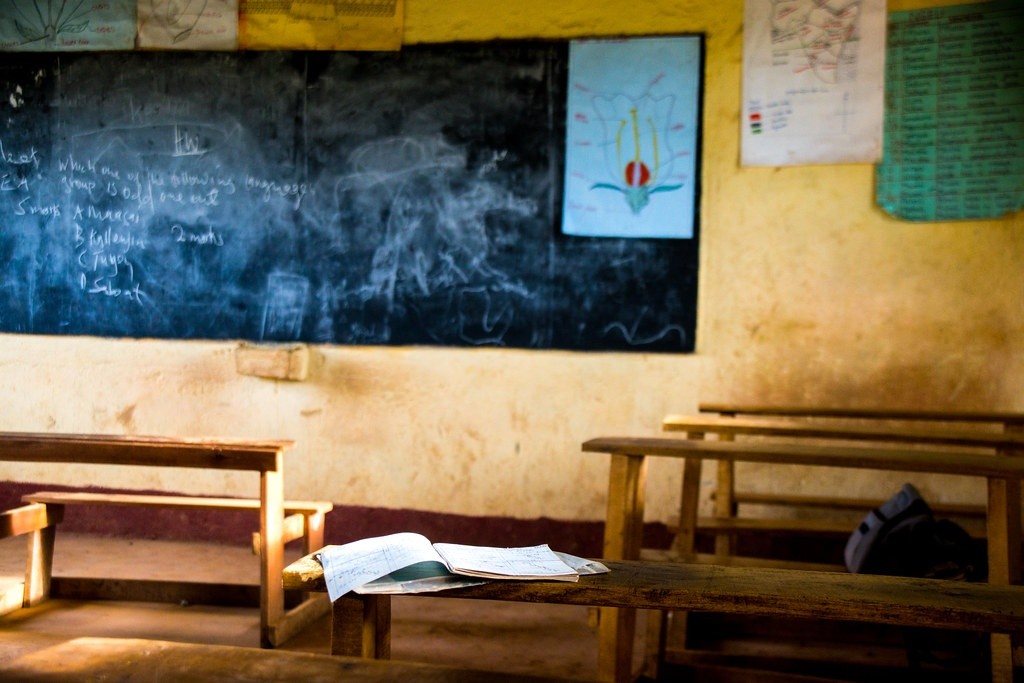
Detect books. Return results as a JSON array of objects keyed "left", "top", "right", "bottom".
[{"left": 320, "top": 532, "right": 580, "bottom": 603}]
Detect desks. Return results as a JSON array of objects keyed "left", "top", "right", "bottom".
[
  {"left": 0, "top": 431, "right": 333, "bottom": 649},
  {"left": 700, "top": 402, "right": 1024, "bottom": 568},
  {"left": 661, "top": 413, "right": 1024, "bottom": 650},
  {"left": 582, "top": 435, "right": 1024, "bottom": 683}
]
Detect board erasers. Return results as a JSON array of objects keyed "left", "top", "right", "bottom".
[{"left": 236, "top": 344, "right": 309, "bottom": 382}]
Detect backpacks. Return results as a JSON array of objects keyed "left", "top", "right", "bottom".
[{"left": 845, "top": 483, "right": 989, "bottom": 577}]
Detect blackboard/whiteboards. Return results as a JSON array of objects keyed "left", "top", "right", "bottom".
[{"left": 0, "top": 29, "right": 707, "bottom": 355}]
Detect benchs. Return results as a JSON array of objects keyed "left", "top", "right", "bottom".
[
  {"left": 281, "top": 542, "right": 1024, "bottom": 683},
  {"left": 0, "top": 635, "right": 600, "bottom": 683},
  {"left": 21, "top": 493, "right": 333, "bottom": 515}
]
